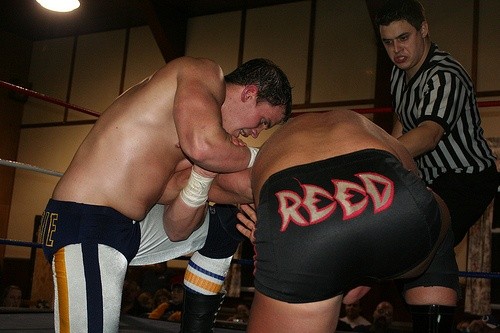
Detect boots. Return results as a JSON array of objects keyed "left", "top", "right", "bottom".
[
  {"left": 405, "top": 303, "right": 461, "bottom": 333},
  {"left": 179, "top": 285, "right": 227, "bottom": 332}
]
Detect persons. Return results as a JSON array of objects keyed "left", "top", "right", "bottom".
[
  {"left": 121, "top": 277, "right": 184, "bottom": 323},
  {"left": 374, "top": 1, "right": 500, "bottom": 333},
  {"left": 160, "top": 100, "right": 451, "bottom": 333},
  {"left": 0, "top": 285, "right": 22, "bottom": 307},
  {"left": 39, "top": 56, "right": 294, "bottom": 333}
]
